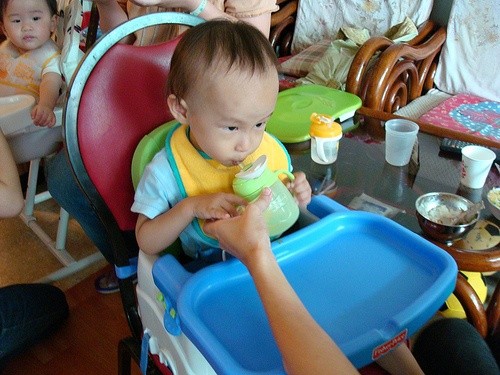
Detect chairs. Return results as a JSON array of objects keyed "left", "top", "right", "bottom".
[{"left": 5, "top": 0, "right": 500, "bottom": 375}]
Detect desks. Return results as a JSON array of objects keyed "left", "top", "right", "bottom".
[{"left": 287, "top": 107, "right": 500, "bottom": 345}]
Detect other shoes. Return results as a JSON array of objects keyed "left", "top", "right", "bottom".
[{"left": 96, "top": 269, "right": 139, "bottom": 294}]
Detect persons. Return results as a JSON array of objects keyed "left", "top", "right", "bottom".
[
  {"left": 0, "top": 128, "right": 73, "bottom": 375},
  {"left": 0, "top": 0, "right": 64, "bottom": 128},
  {"left": 201, "top": 185, "right": 500, "bottom": 375},
  {"left": 128, "top": 18, "right": 312, "bottom": 277},
  {"left": 48, "top": 0, "right": 280, "bottom": 295}
]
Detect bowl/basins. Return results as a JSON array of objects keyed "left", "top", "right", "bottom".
[
  {"left": 487, "top": 188, "right": 500, "bottom": 220},
  {"left": 415, "top": 192, "right": 480, "bottom": 241}
]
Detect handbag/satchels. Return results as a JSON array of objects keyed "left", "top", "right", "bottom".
[{"left": 295, "top": 33, "right": 417, "bottom": 106}]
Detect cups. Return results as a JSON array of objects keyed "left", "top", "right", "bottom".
[
  {"left": 385, "top": 119, "right": 419, "bottom": 167},
  {"left": 310, "top": 113, "right": 342, "bottom": 165},
  {"left": 461, "top": 145, "right": 497, "bottom": 189}
]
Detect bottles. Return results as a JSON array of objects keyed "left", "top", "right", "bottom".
[{"left": 232, "top": 155, "right": 300, "bottom": 236}]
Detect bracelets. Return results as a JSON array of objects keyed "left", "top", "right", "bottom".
[{"left": 190, "top": 0, "right": 206, "bottom": 16}]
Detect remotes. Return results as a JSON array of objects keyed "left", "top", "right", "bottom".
[{"left": 441, "top": 138, "right": 500, "bottom": 164}]
